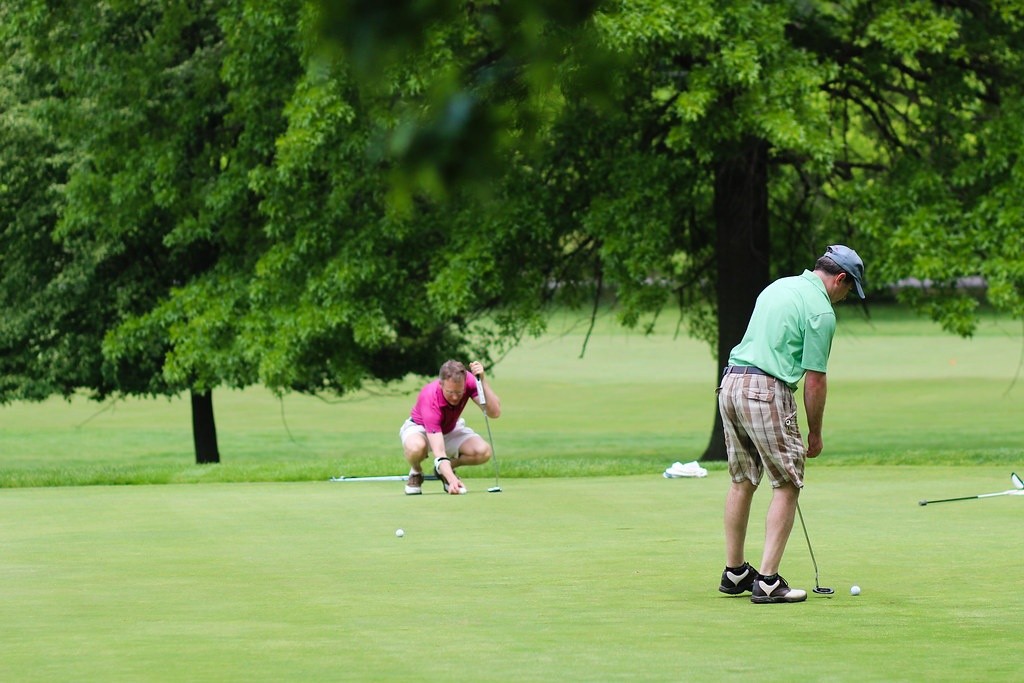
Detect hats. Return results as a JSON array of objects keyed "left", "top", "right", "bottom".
[{"left": 824, "top": 244, "right": 866, "bottom": 299}]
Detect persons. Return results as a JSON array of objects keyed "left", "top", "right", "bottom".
[
  {"left": 399, "top": 359, "right": 500, "bottom": 495},
  {"left": 714, "top": 245, "right": 866, "bottom": 604}
]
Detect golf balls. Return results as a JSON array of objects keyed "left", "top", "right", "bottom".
[
  {"left": 850, "top": 586, "right": 861, "bottom": 596},
  {"left": 395, "top": 528, "right": 405, "bottom": 538},
  {"left": 459, "top": 487, "right": 467, "bottom": 495}
]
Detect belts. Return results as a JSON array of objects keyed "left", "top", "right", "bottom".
[
  {"left": 409, "top": 416, "right": 415, "bottom": 423},
  {"left": 723, "top": 365, "right": 770, "bottom": 374}
]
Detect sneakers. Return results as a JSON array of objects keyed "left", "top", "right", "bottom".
[
  {"left": 719, "top": 561, "right": 760, "bottom": 598},
  {"left": 434, "top": 466, "right": 466, "bottom": 493},
  {"left": 751, "top": 572, "right": 807, "bottom": 603},
  {"left": 405, "top": 466, "right": 424, "bottom": 495}
]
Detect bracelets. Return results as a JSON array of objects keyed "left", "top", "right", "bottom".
[{"left": 434, "top": 457, "right": 451, "bottom": 473}]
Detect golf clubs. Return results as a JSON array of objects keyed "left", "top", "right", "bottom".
[
  {"left": 795, "top": 502, "right": 836, "bottom": 596},
  {"left": 918, "top": 471, "right": 1024, "bottom": 507},
  {"left": 473, "top": 373, "right": 504, "bottom": 494}
]
